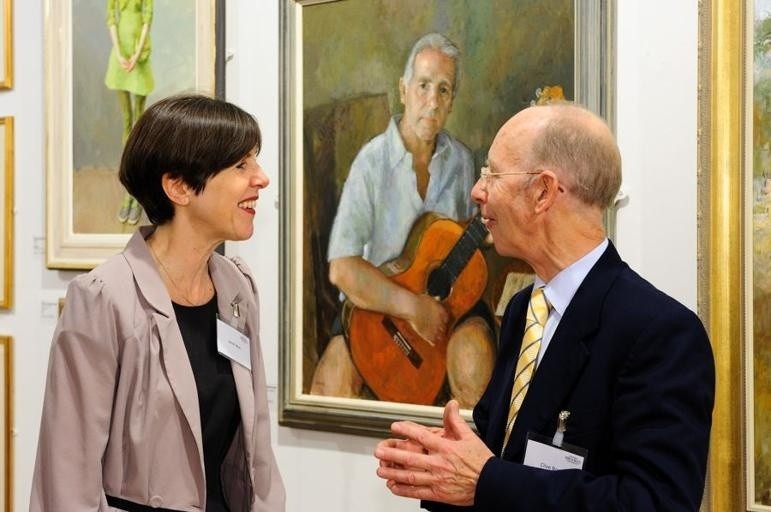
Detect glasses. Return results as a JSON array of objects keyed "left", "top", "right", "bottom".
[{"left": 480, "top": 165, "right": 565, "bottom": 196}]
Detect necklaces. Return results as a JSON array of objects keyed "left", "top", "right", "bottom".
[{"left": 147, "top": 237, "right": 209, "bottom": 306}]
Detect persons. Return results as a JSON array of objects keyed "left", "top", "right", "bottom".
[
  {"left": 27, "top": 90, "right": 289, "bottom": 512},
  {"left": 306, "top": 30, "right": 501, "bottom": 410},
  {"left": 373, "top": 102, "right": 719, "bottom": 512},
  {"left": 102, "top": 0, "right": 155, "bottom": 228}
]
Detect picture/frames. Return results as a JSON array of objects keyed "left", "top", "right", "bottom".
[
  {"left": 697, "top": 0, "right": 771, "bottom": 511},
  {"left": 276, "top": 2, "right": 617, "bottom": 444},
  {"left": 38, "top": 0, "right": 228, "bottom": 274},
  {"left": 1, "top": 0, "right": 12, "bottom": 512}
]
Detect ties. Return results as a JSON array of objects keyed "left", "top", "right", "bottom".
[{"left": 499, "top": 287, "right": 554, "bottom": 459}]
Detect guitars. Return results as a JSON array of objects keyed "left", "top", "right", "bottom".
[{"left": 341, "top": 211, "right": 490, "bottom": 405}]
